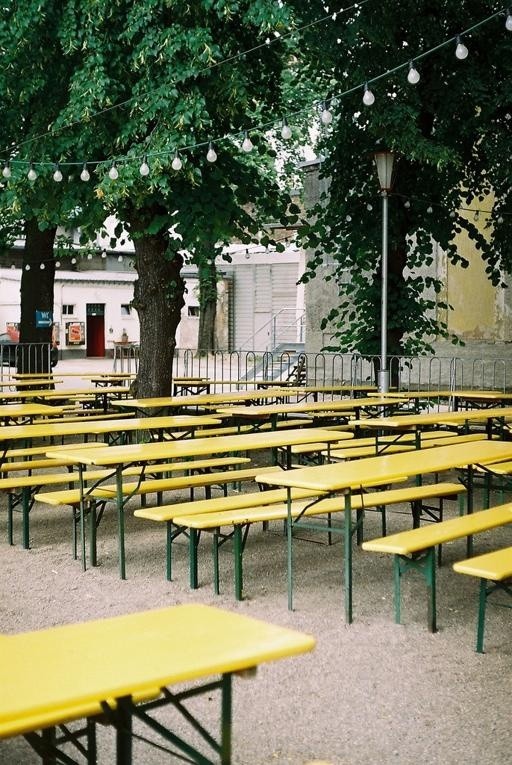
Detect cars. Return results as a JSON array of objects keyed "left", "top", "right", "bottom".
[{"left": 0, "top": 331, "right": 58, "bottom": 367}]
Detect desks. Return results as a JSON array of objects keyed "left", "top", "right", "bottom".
[{"left": 1, "top": 600, "right": 316, "bottom": 764}]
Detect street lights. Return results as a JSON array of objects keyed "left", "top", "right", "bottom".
[{"left": 372, "top": 137, "right": 404, "bottom": 435}]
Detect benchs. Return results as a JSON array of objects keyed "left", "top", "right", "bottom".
[{"left": 1, "top": 373, "right": 511, "bottom": 653}]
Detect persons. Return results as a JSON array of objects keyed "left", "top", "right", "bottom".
[{"left": 6, "top": 323, "right": 19, "bottom": 342}]
